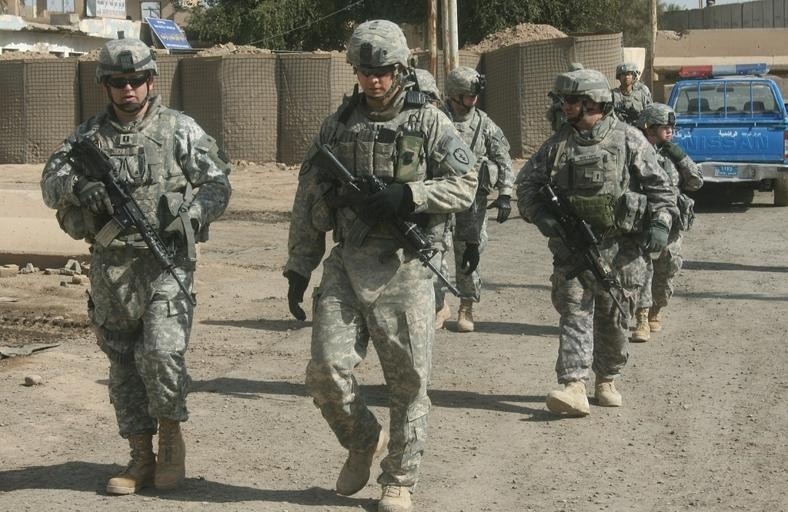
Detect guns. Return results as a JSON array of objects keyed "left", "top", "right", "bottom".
[
  {"left": 539, "top": 183, "right": 630, "bottom": 321},
  {"left": 613, "top": 105, "right": 640, "bottom": 120},
  {"left": 309, "top": 146, "right": 463, "bottom": 297},
  {"left": 73, "top": 138, "right": 198, "bottom": 307}
]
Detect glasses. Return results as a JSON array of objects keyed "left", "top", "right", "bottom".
[
  {"left": 358, "top": 65, "right": 392, "bottom": 79},
  {"left": 563, "top": 94, "right": 579, "bottom": 105},
  {"left": 108, "top": 76, "right": 147, "bottom": 89}
]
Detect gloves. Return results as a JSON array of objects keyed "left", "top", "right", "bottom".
[
  {"left": 460, "top": 244, "right": 479, "bottom": 274},
  {"left": 488, "top": 196, "right": 512, "bottom": 223},
  {"left": 283, "top": 270, "right": 307, "bottom": 322},
  {"left": 365, "top": 182, "right": 414, "bottom": 221},
  {"left": 532, "top": 210, "right": 564, "bottom": 240},
  {"left": 163, "top": 217, "right": 200, "bottom": 245},
  {"left": 663, "top": 143, "right": 686, "bottom": 161},
  {"left": 640, "top": 219, "right": 669, "bottom": 255},
  {"left": 73, "top": 178, "right": 115, "bottom": 214}
]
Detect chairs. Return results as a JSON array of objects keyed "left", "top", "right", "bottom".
[
  {"left": 743, "top": 100, "right": 766, "bottom": 114},
  {"left": 686, "top": 96, "right": 713, "bottom": 113}
]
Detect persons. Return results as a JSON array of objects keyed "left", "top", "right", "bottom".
[
  {"left": 611, "top": 63, "right": 653, "bottom": 126},
  {"left": 630, "top": 102, "right": 704, "bottom": 343},
  {"left": 280, "top": 18, "right": 483, "bottom": 512},
  {"left": 546, "top": 63, "right": 584, "bottom": 133},
  {"left": 433, "top": 65, "right": 514, "bottom": 333},
  {"left": 39, "top": 35, "right": 233, "bottom": 496},
  {"left": 516, "top": 68, "right": 674, "bottom": 418}
]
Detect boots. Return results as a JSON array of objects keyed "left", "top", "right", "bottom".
[
  {"left": 546, "top": 382, "right": 590, "bottom": 418},
  {"left": 456, "top": 298, "right": 474, "bottom": 332},
  {"left": 153, "top": 419, "right": 186, "bottom": 491},
  {"left": 108, "top": 431, "right": 156, "bottom": 495},
  {"left": 593, "top": 376, "right": 621, "bottom": 407},
  {"left": 629, "top": 307, "right": 662, "bottom": 343},
  {"left": 435, "top": 300, "right": 451, "bottom": 331}
]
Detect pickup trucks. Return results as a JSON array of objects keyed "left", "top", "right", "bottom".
[{"left": 656, "top": 62, "right": 788, "bottom": 208}]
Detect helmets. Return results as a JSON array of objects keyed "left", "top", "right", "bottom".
[
  {"left": 94, "top": 40, "right": 159, "bottom": 85},
  {"left": 345, "top": 20, "right": 412, "bottom": 70},
  {"left": 445, "top": 66, "right": 483, "bottom": 97},
  {"left": 636, "top": 102, "right": 678, "bottom": 130},
  {"left": 615, "top": 62, "right": 639, "bottom": 79},
  {"left": 555, "top": 68, "right": 613, "bottom": 105}
]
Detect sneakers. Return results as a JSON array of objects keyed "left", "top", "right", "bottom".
[
  {"left": 378, "top": 483, "right": 412, "bottom": 512},
  {"left": 336, "top": 431, "right": 387, "bottom": 495}
]
